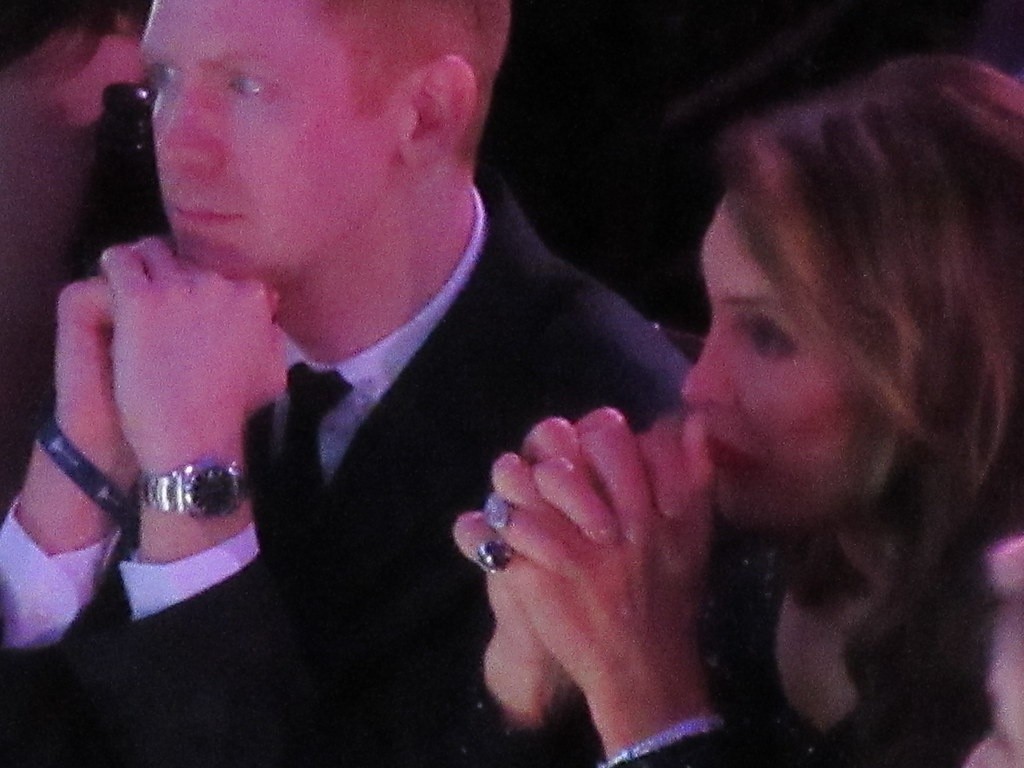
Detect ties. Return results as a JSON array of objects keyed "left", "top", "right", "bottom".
[{"left": 278, "top": 362, "right": 351, "bottom": 532}]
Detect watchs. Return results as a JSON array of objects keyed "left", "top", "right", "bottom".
[{"left": 144, "top": 459, "right": 250, "bottom": 518}]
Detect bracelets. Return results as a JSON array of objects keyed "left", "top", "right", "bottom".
[{"left": 35, "top": 423, "right": 130, "bottom": 524}]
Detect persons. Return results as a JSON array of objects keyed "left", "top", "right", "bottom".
[
  {"left": 0, "top": 1, "right": 692, "bottom": 768},
  {"left": 453, "top": 55, "right": 1023, "bottom": 767}
]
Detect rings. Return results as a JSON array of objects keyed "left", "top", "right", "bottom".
[
  {"left": 484, "top": 498, "right": 513, "bottom": 525},
  {"left": 479, "top": 542, "right": 513, "bottom": 572}
]
